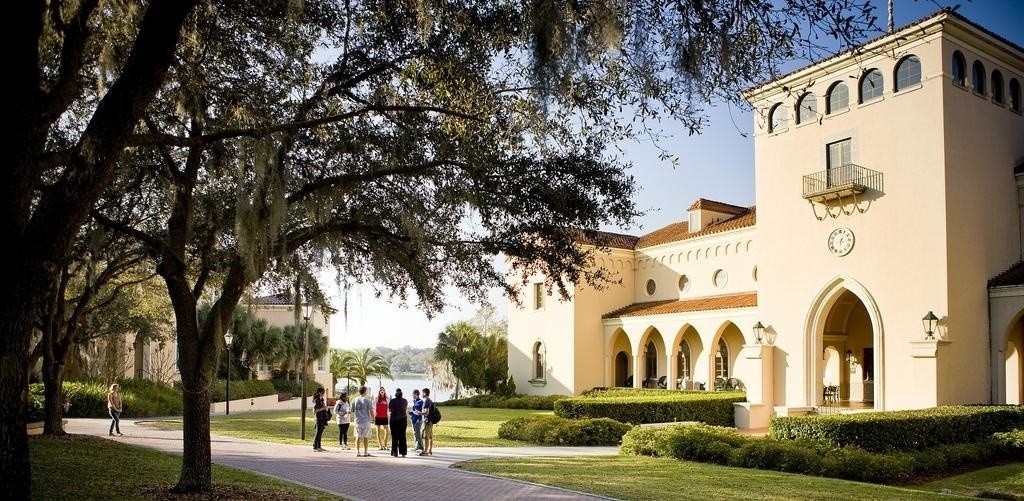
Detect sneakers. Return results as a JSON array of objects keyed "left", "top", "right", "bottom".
[
  {"left": 108, "top": 432, "right": 115, "bottom": 436},
  {"left": 357, "top": 454, "right": 371, "bottom": 457},
  {"left": 312, "top": 446, "right": 325, "bottom": 452},
  {"left": 116, "top": 433, "right": 123, "bottom": 436},
  {"left": 339, "top": 445, "right": 350, "bottom": 450},
  {"left": 412, "top": 447, "right": 432, "bottom": 456}
]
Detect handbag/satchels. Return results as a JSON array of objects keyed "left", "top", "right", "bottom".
[{"left": 432, "top": 407, "right": 441, "bottom": 423}]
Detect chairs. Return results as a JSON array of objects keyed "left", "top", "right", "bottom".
[
  {"left": 824, "top": 385, "right": 837, "bottom": 403},
  {"left": 643, "top": 375, "right": 667, "bottom": 388},
  {"left": 715, "top": 377, "right": 739, "bottom": 391}
]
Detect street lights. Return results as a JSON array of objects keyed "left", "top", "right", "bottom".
[
  {"left": 222, "top": 327, "right": 234, "bottom": 416},
  {"left": 346, "top": 363, "right": 351, "bottom": 404},
  {"left": 300, "top": 297, "right": 314, "bottom": 441}
]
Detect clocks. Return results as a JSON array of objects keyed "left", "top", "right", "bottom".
[{"left": 828, "top": 225, "right": 854, "bottom": 259}]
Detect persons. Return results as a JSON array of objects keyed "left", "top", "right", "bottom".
[
  {"left": 332, "top": 392, "right": 352, "bottom": 450},
  {"left": 373, "top": 387, "right": 390, "bottom": 450},
  {"left": 349, "top": 386, "right": 375, "bottom": 456},
  {"left": 388, "top": 387, "right": 408, "bottom": 457},
  {"left": 107, "top": 383, "right": 124, "bottom": 436},
  {"left": 416, "top": 388, "right": 434, "bottom": 457},
  {"left": 408, "top": 390, "right": 424, "bottom": 450},
  {"left": 311, "top": 387, "right": 330, "bottom": 452}
]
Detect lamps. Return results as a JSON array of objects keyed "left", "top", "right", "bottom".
[
  {"left": 846, "top": 350, "right": 853, "bottom": 363},
  {"left": 753, "top": 321, "right": 765, "bottom": 345},
  {"left": 923, "top": 310, "right": 939, "bottom": 340}
]
